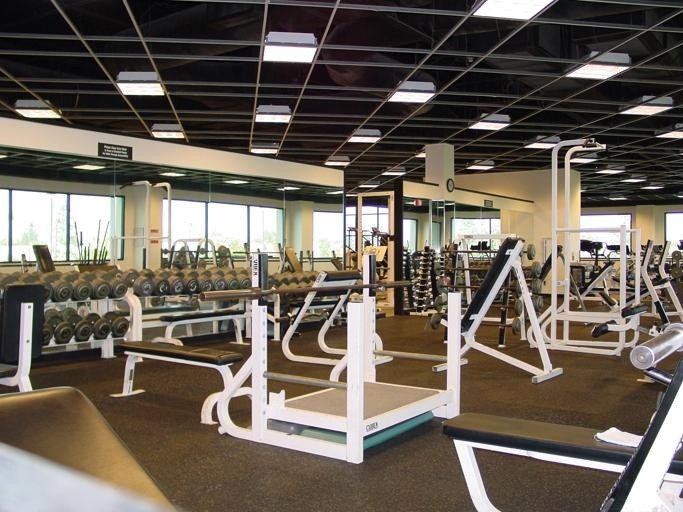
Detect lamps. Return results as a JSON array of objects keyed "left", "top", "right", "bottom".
[
  {"left": 256, "top": 105, "right": 292, "bottom": 124},
  {"left": 386, "top": 80, "right": 435, "bottom": 104},
  {"left": 594, "top": 165, "right": 626, "bottom": 175},
  {"left": 358, "top": 180, "right": 380, "bottom": 188},
  {"left": 470, "top": 0, "right": 557, "bottom": 22},
  {"left": 569, "top": 152, "right": 598, "bottom": 164},
  {"left": 262, "top": 31, "right": 317, "bottom": 63},
  {"left": 380, "top": 166, "right": 407, "bottom": 176},
  {"left": 415, "top": 151, "right": 426, "bottom": 158},
  {"left": 277, "top": 184, "right": 301, "bottom": 192},
  {"left": 615, "top": 95, "right": 676, "bottom": 116},
  {"left": 639, "top": 182, "right": 664, "bottom": 190},
  {"left": 468, "top": 113, "right": 511, "bottom": 130},
  {"left": 618, "top": 174, "right": 647, "bottom": 184},
  {"left": 467, "top": 160, "right": 496, "bottom": 172},
  {"left": 117, "top": 68, "right": 183, "bottom": 140},
  {"left": 565, "top": 50, "right": 630, "bottom": 82},
  {"left": 250, "top": 142, "right": 280, "bottom": 156},
  {"left": 523, "top": 135, "right": 561, "bottom": 150},
  {"left": 325, "top": 189, "right": 361, "bottom": 199},
  {"left": 653, "top": 122, "right": 683, "bottom": 139},
  {"left": 16, "top": 98, "right": 61, "bottom": 121},
  {"left": 347, "top": 128, "right": 382, "bottom": 143},
  {"left": 324, "top": 155, "right": 350, "bottom": 167}
]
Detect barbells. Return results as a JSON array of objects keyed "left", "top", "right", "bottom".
[
  {"left": 161, "top": 246, "right": 229, "bottom": 268},
  {"left": 430, "top": 244, "right": 544, "bottom": 335}
]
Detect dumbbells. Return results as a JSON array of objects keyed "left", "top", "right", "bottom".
[
  {"left": 40, "top": 308, "right": 129, "bottom": 342},
  {"left": 267, "top": 271, "right": 317, "bottom": 288},
  {"left": 412, "top": 251, "right": 435, "bottom": 309},
  {"left": 0, "top": 268, "right": 252, "bottom": 299}
]
